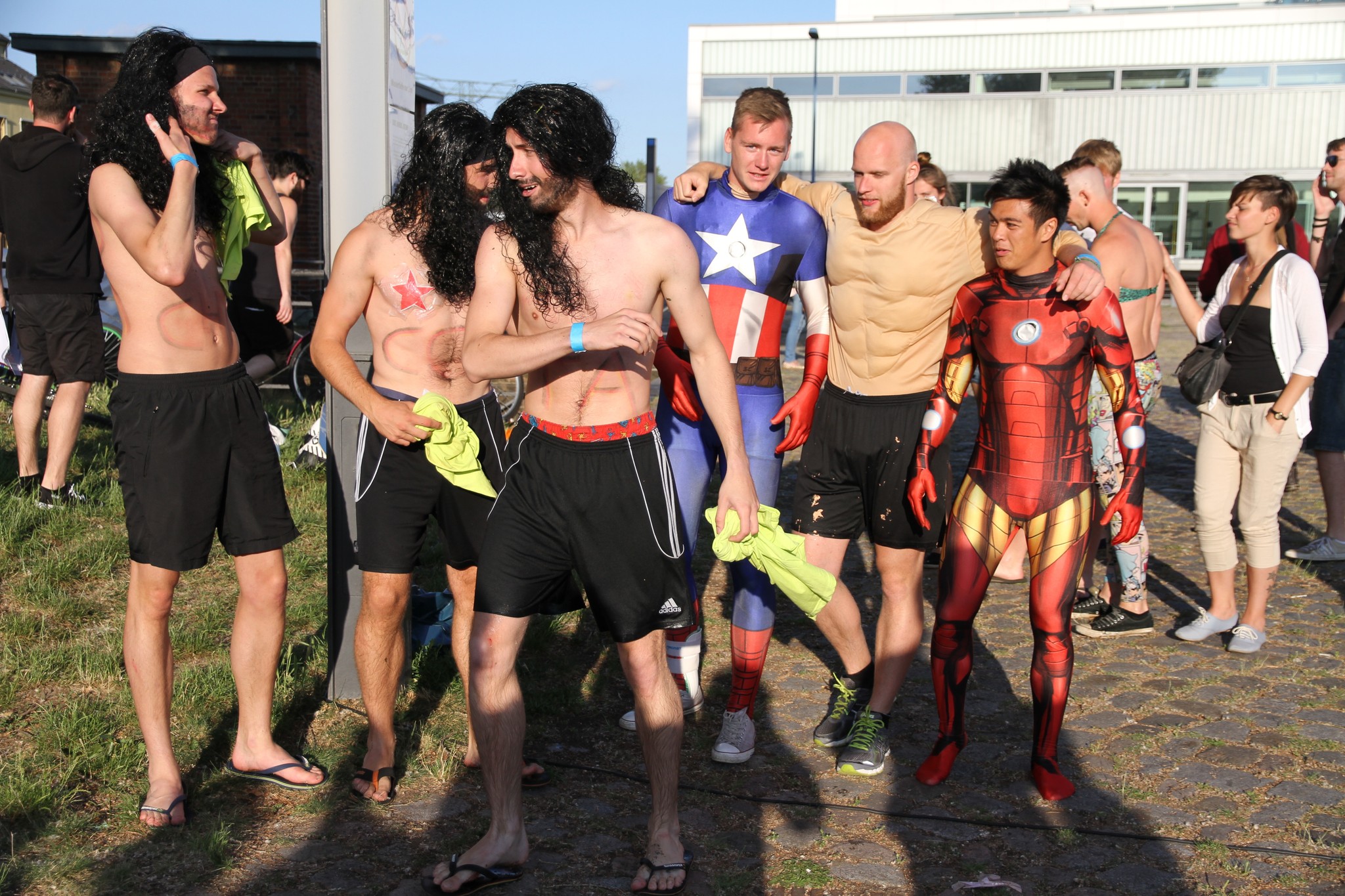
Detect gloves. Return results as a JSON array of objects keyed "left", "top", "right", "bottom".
[
  {"left": 771, "top": 382, "right": 819, "bottom": 454},
  {"left": 654, "top": 347, "right": 704, "bottom": 422},
  {"left": 1100, "top": 477, "right": 1143, "bottom": 545},
  {"left": 907, "top": 469, "right": 938, "bottom": 531}
]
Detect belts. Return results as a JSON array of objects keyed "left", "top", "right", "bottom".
[{"left": 1219, "top": 390, "right": 1283, "bottom": 408}]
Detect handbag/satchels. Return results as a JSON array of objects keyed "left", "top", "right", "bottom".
[{"left": 1175, "top": 345, "right": 1231, "bottom": 405}]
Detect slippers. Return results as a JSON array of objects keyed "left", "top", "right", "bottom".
[
  {"left": 990, "top": 576, "right": 1028, "bottom": 583},
  {"left": 350, "top": 748, "right": 398, "bottom": 806},
  {"left": 140, "top": 779, "right": 191, "bottom": 830},
  {"left": 1073, "top": 587, "right": 1096, "bottom": 605},
  {"left": 634, "top": 850, "right": 694, "bottom": 896},
  {"left": 521, "top": 755, "right": 552, "bottom": 789},
  {"left": 421, "top": 852, "right": 523, "bottom": 896},
  {"left": 225, "top": 754, "right": 328, "bottom": 790}
]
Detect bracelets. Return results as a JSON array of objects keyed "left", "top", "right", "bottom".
[
  {"left": 571, "top": 322, "right": 587, "bottom": 354},
  {"left": 1314, "top": 217, "right": 1329, "bottom": 221},
  {"left": 1311, "top": 234, "right": 1325, "bottom": 243},
  {"left": 1312, "top": 224, "right": 1329, "bottom": 228},
  {"left": 170, "top": 153, "right": 200, "bottom": 175},
  {"left": 1073, "top": 253, "right": 1102, "bottom": 269}
]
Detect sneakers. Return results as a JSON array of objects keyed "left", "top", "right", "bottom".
[
  {"left": 264, "top": 410, "right": 290, "bottom": 446},
  {"left": 1075, "top": 606, "right": 1154, "bottom": 638},
  {"left": 624, "top": 684, "right": 704, "bottom": 730},
  {"left": 32, "top": 486, "right": 105, "bottom": 513},
  {"left": 713, "top": 707, "right": 756, "bottom": 763},
  {"left": 1174, "top": 607, "right": 1240, "bottom": 642},
  {"left": 1228, "top": 624, "right": 1267, "bottom": 653},
  {"left": 1284, "top": 534, "right": 1345, "bottom": 561},
  {"left": 11, "top": 481, "right": 39, "bottom": 501},
  {"left": 837, "top": 704, "right": 891, "bottom": 776},
  {"left": 1071, "top": 595, "right": 1114, "bottom": 619},
  {"left": 814, "top": 672, "right": 873, "bottom": 748}
]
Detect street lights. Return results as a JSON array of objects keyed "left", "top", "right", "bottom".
[{"left": 808, "top": 27, "right": 820, "bottom": 184}]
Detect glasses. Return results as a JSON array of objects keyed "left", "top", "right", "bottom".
[{"left": 1325, "top": 155, "right": 1339, "bottom": 167}]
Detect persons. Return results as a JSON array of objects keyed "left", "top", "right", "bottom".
[
  {"left": 418, "top": 84, "right": 760, "bottom": 895},
  {"left": 221, "top": 151, "right": 311, "bottom": 381},
  {"left": 906, "top": 157, "right": 1150, "bottom": 802},
  {"left": 914, "top": 152, "right": 957, "bottom": 207},
  {"left": 618, "top": 87, "right": 831, "bottom": 766},
  {"left": 1161, "top": 173, "right": 1329, "bottom": 651},
  {"left": 80, "top": 26, "right": 327, "bottom": 829},
  {"left": 1198, "top": 208, "right": 1312, "bottom": 491},
  {"left": 675, "top": 120, "right": 1104, "bottom": 779},
  {"left": 0, "top": 71, "right": 108, "bottom": 513},
  {"left": 309, "top": 100, "right": 547, "bottom": 806},
  {"left": 1278, "top": 136, "right": 1345, "bottom": 561},
  {"left": 990, "top": 139, "right": 1165, "bottom": 639}
]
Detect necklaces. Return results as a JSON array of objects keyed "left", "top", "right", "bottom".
[{"left": 1244, "top": 256, "right": 1270, "bottom": 282}]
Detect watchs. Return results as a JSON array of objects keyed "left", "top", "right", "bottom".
[{"left": 1269, "top": 408, "right": 1290, "bottom": 421}]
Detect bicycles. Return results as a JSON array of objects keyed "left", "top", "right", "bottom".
[{"left": 285, "top": 331, "right": 327, "bottom": 414}]
